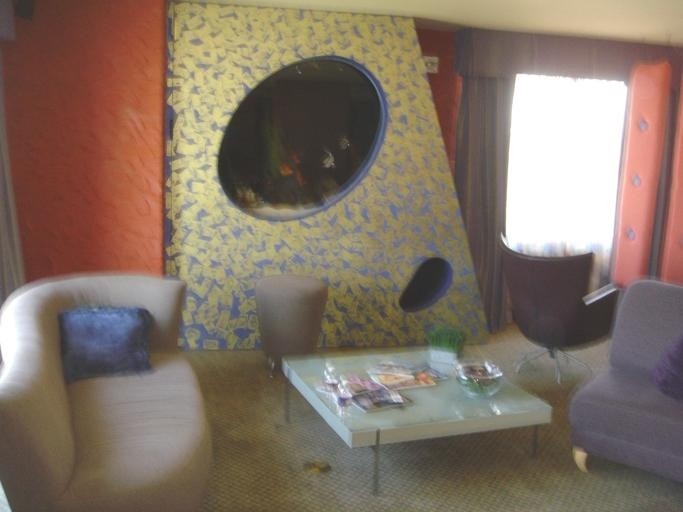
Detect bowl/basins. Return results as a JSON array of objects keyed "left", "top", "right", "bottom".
[{"left": 454, "top": 362, "right": 506, "bottom": 399}]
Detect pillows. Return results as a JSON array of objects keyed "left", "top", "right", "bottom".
[{"left": 56, "top": 305, "right": 152, "bottom": 385}]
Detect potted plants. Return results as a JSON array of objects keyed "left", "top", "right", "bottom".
[{"left": 425, "top": 327, "right": 463, "bottom": 365}]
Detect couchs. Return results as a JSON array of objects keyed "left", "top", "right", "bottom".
[{"left": 0, "top": 273, "right": 212, "bottom": 512}]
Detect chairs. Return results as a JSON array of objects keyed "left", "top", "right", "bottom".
[
  {"left": 568, "top": 281, "right": 683, "bottom": 486},
  {"left": 498, "top": 232, "right": 625, "bottom": 383}
]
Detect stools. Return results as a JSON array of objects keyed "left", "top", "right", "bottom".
[{"left": 254, "top": 272, "right": 330, "bottom": 383}]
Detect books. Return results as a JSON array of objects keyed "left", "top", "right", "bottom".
[{"left": 316, "top": 359, "right": 448, "bottom": 414}]
